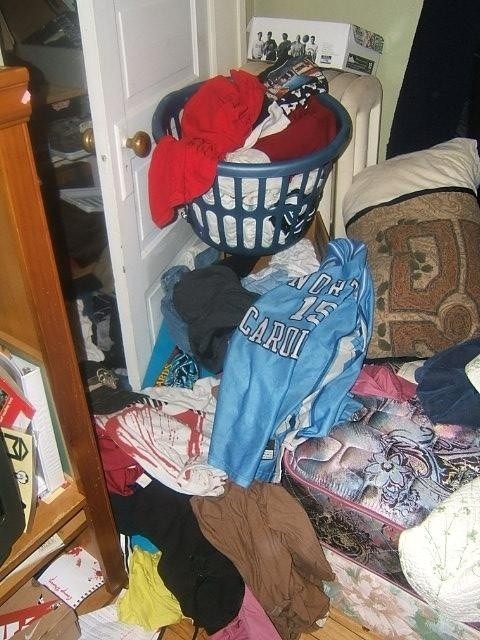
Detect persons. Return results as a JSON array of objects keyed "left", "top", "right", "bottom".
[{"left": 251, "top": 31, "right": 318, "bottom": 64}]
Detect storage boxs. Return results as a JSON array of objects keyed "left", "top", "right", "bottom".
[{"left": 246, "top": 18, "right": 386, "bottom": 76}]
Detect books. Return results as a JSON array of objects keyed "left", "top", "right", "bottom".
[{"left": 59, "top": 186, "right": 104, "bottom": 214}]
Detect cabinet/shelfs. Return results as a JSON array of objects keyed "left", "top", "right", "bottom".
[{"left": 0, "top": 65, "right": 128, "bottom": 640}]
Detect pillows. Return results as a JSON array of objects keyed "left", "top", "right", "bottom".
[{"left": 342, "top": 137, "right": 479, "bottom": 361}]
